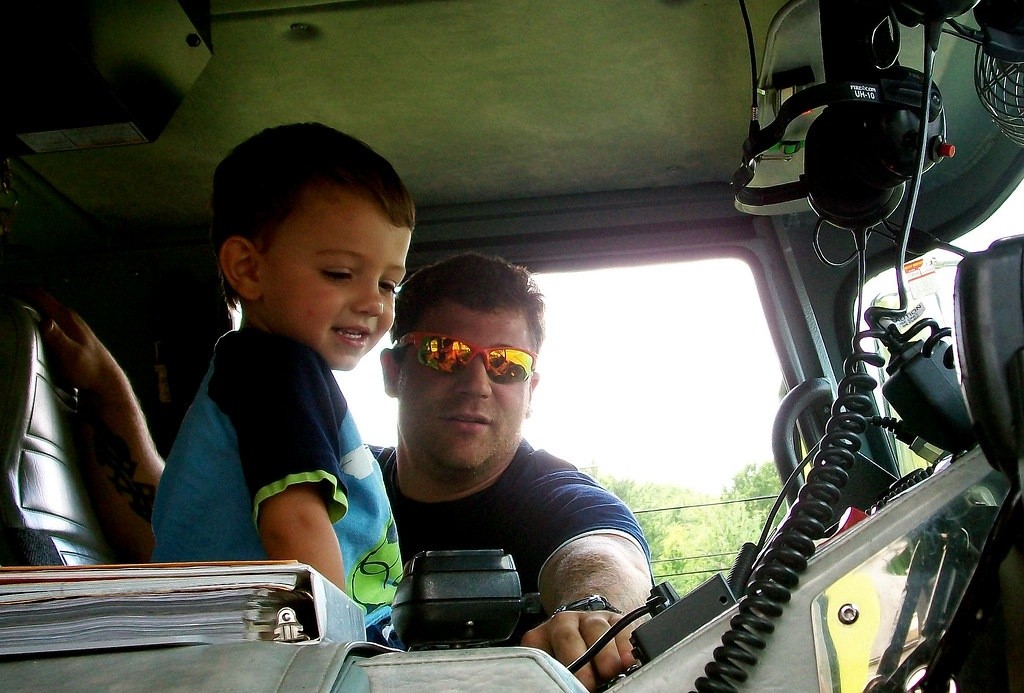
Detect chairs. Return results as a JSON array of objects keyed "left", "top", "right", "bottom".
[{"left": 0, "top": 293, "right": 588, "bottom": 693}]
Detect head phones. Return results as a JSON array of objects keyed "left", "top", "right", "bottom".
[{"left": 730, "top": 65, "right": 955, "bottom": 232}]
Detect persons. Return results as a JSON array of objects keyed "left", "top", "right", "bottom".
[
  {"left": 153, "top": 122, "right": 416, "bottom": 649},
  {"left": 422, "top": 337, "right": 458, "bottom": 371},
  {"left": 488, "top": 354, "right": 521, "bottom": 383},
  {"left": 7, "top": 252, "right": 657, "bottom": 693}
]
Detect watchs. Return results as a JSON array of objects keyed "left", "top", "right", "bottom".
[{"left": 551, "top": 594, "right": 622, "bottom": 620}]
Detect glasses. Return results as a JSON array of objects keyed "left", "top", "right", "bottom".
[{"left": 398, "top": 332, "right": 537, "bottom": 383}]
